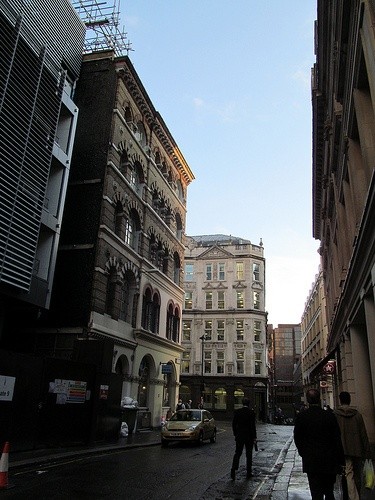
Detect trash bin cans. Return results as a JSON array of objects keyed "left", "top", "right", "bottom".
[
  {"left": 137, "top": 407, "right": 151, "bottom": 428},
  {"left": 121, "top": 405, "right": 138, "bottom": 427}
]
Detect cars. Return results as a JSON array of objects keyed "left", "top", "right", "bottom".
[{"left": 160, "top": 409, "right": 217, "bottom": 449}]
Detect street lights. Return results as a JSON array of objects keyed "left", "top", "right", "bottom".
[{"left": 199, "top": 334, "right": 206, "bottom": 409}]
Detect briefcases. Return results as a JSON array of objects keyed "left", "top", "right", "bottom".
[{"left": 339, "top": 473, "right": 349, "bottom": 500}]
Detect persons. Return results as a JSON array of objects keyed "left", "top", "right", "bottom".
[
  {"left": 230, "top": 398, "right": 259, "bottom": 482},
  {"left": 332, "top": 391, "right": 370, "bottom": 500},
  {"left": 293, "top": 388, "right": 345, "bottom": 500},
  {"left": 176, "top": 398, "right": 194, "bottom": 412},
  {"left": 325, "top": 404, "right": 334, "bottom": 414}
]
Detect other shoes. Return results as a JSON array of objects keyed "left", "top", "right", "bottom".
[
  {"left": 247, "top": 473, "right": 254, "bottom": 478},
  {"left": 231, "top": 468, "right": 235, "bottom": 480}
]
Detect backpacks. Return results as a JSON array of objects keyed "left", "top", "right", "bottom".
[{"left": 177, "top": 404, "right": 183, "bottom": 410}]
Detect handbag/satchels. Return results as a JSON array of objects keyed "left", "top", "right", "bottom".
[
  {"left": 361, "top": 459, "right": 375, "bottom": 491},
  {"left": 254, "top": 440, "right": 258, "bottom": 451}
]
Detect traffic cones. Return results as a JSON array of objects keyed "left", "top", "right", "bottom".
[{"left": 0, "top": 441, "right": 11, "bottom": 490}]
